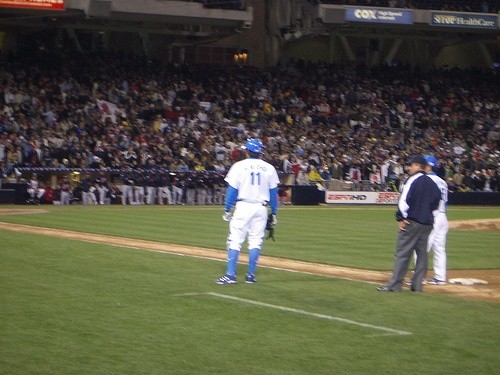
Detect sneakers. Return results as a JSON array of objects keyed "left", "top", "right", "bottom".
[
  {"left": 216, "top": 274, "right": 237, "bottom": 284},
  {"left": 245, "top": 274, "right": 256, "bottom": 284}
]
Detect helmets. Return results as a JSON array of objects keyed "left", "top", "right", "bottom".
[
  {"left": 424, "top": 155, "right": 438, "bottom": 167},
  {"left": 246, "top": 138, "right": 262, "bottom": 153}
]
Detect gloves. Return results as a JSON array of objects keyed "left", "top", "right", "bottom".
[
  {"left": 271, "top": 215, "right": 277, "bottom": 225},
  {"left": 222, "top": 211, "right": 231, "bottom": 222}
]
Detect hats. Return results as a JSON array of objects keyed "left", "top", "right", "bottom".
[{"left": 405, "top": 155, "right": 426, "bottom": 164}]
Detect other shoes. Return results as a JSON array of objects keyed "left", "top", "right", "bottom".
[
  {"left": 405, "top": 281, "right": 426, "bottom": 286},
  {"left": 428, "top": 278, "right": 446, "bottom": 285},
  {"left": 377, "top": 286, "right": 392, "bottom": 291}
]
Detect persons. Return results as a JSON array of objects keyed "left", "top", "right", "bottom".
[
  {"left": 377, "top": 155, "right": 441, "bottom": 294},
  {"left": 402, "top": 155, "right": 449, "bottom": 286},
  {"left": 215, "top": 137, "right": 281, "bottom": 285},
  {"left": 0, "top": 55, "right": 500, "bottom": 204}
]
